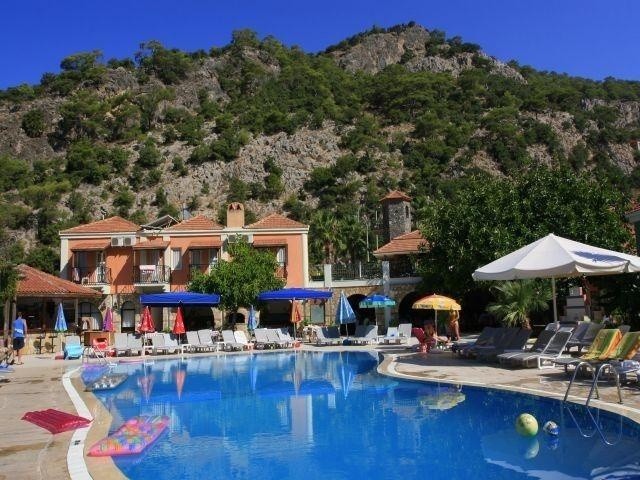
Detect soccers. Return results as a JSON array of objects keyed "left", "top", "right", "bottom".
[
  {"left": 516, "top": 413, "right": 538, "bottom": 436},
  {"left": 544, "top": 421, "right": 559, "bottom": 438}
]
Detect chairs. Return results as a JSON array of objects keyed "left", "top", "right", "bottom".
[
  {"left": 308, "top": 323, "right": 453, "bottom": 354},
  {"left": 64, "top": 334, "right": 86, "bottom": 360},
  {"left": 113, "top": 327, "right": 297, "bottom": 358},
  {"left": 454, "top": 324, "right": 640, "bottom": 385}
]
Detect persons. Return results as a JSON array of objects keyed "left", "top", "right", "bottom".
[
  {"left": 445, "top": 308, "right": 462, "bottom": 350},
  {"left": 10, "top": 310, "right": 30, "bottom": 366},
  {"left": 423, "top": 320, "right": 447, "bottom": 343}
]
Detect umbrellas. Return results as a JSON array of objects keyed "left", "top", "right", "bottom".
[
  {"left": 359, "top": 292, "right": 397, "bottom": 325},
  {"left": 54, "top": 301, "right": 69, "bottom": 355},
  {"left": 141, "top": 361, "right": 156, "bottom": 403},
  {"left": 247, "top": 303, "right": 257, "bottom": 337},
  {"left": 336, "top": 290, "right": 358, "bottom": 338},
  {"left": 412, "top": 292, "right": 462, "bottom": 332},
  {"left": 173, "top": 307, "right": 188, "bottom": 346},
  {"left": 248, "top": 353, "right": 258, "bottom": 393},
  {"left": 138, "top": 305, "right": 156, "bottom": 347},
  {"left": 102, "top": 304, "right": 116, "bottom": 349},
  {"left": 290, "top": 299, "right": 302, "bottom": 338},
  {"left": 257, "top": 288, "right": 332, "bottom": 300},
  {"left": 422, "top": 381, "right": 465, "bottom": 412},
  {"left": 176, "top": 357, "right": 187, "bottom": 399},
  {"left": 337, "top": 351, "right": 357, "bottom": 401},
  {"left": 471, "top": 230, "right": 640, "bottom": 323},
  {"left": 294, "top": 351, "right": 303, "bottom": 393},
  {"left": 140, "top": 290, "right": 221, "bottom": 306}
]
